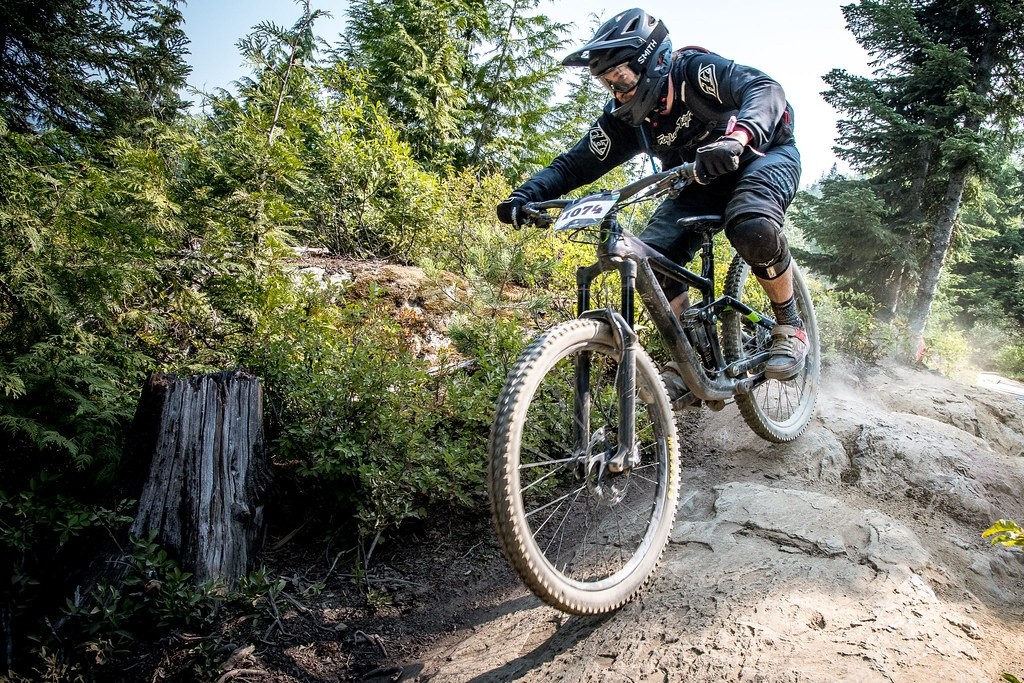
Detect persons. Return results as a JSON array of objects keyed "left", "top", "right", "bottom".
[{"left": 495, "top": 5, "right": 811, "bottom": 411}]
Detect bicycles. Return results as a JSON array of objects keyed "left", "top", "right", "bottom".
[{"left": 484, "top": 160, "right": 822, "bottom": 620}]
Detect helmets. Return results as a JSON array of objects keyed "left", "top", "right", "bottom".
[{"left": 561, "top": 8, "right": 672, "bottom": 127}]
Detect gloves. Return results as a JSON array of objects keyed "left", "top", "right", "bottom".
[
  {"left": 693, "top": 136, "right": 745, "bottom": 185},
  {"left": 497, "top": 189, "right": 528, "bottom": 231}
]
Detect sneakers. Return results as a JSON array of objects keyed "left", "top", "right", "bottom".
[
  {"left": 765, "top": 319, "right": 810, "bottom": 379},
  {"left": 659, "top": 361, "right": 701, "bottom": 411}
]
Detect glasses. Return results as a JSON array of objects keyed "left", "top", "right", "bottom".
[{"left": 594, "top": 18, "right": 670, "bottom": 99}]
